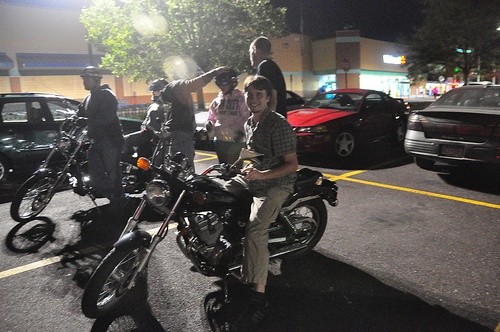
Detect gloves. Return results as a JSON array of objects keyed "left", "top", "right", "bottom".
[{"left": 74, "top": 117, "right": 90, "bottom": 125}]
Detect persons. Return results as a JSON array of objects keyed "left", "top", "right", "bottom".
[
  {"left": 206, "top": 69, "right": 251, "bottom": 168},
  {"left": 158, "top": 62, "right": 233, "bottom": 174},
  {"left": 319, "top": 85, "right": 327, "bottom": 93},
  {"left": 232, "top": 75, "right": 300, "bottom": 328},
  {"left": 249, "top": 36, "right": 287, "bottom": 118},
  {"left": 77, "top": 64, "right": 126, "bottom": 218},
  {"left": 431, "top": 86, "right": 440, "bottom": 98},
  {"left": 124, "top": 79, "right": 169, "bottom": 155}
]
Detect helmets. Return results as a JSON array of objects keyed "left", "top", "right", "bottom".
[
  {"left": 80, "top": 65, "right": 102, "bottom": 79},
  {"left": 148, "top": 78, "right": 169, "bottom": 104},
  {"left": 214, "top": 69, "right": 238, "bottom": 83}
]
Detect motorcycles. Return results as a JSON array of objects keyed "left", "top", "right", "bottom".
[
  {"left": 10, "top": 108, "right": 168, "bottom": 223},
  {"left": 82, "top": 103, "right": 340, "bottom": 320}
]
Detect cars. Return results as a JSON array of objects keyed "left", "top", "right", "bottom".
[
  {"left": 195, "top": 88, "right": 308, "bottom": 147},
  {"left": 287, "top": 88, "right": 405, "bottom": 157},
  {"left": 463, "top": 80, "right": 491, "bottom": 87},
  {"left": 405, "top": 86, "right": 497, "bottom": 176},
  {"left": 0, "top": 92, "right": 144, "bottom": 195}
]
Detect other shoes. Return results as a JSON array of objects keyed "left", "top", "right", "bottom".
[{"left": 239, "top": 290, "right": 272, "bottom": 314}]
